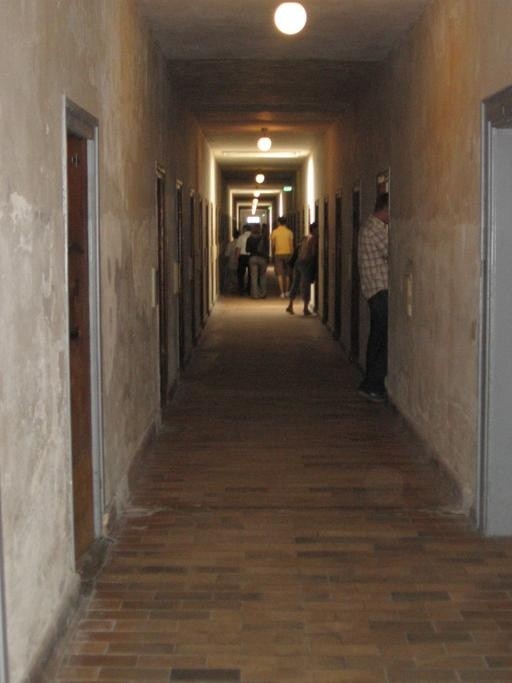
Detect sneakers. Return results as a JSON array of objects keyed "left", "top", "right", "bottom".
[
  {"left": 358, "top": 389, "right": 387, "bottom": 403},
  {"left": 286, "top": 307, "right": 311, "bottom": 315},
  {"left": 280, "top": 291, "right": 290, "bottom": 298}
]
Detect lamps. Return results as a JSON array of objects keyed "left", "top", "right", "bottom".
[
  {"left": 253, "top": 187, "right": 260, "bottom": 198},
  {"left": 257, "top": 128, "right": 272, "bottom": 152},
  {"left": 255, "top": 169, "right": 265, "bottom": 184},
  {"left": 274, "top": 2, "right": 307, "bottom": 35}
]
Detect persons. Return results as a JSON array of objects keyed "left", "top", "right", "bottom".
[
  {"left": 234, "top": 223, "right": 253, "bottom": 296},
  {"left": 245, "top": 222, "right": 268, "bottom": 298},
  {"left": 223, "top": 229, "right": 240, "bottom": 294},
  {"left": 354, "top": 191, "right": 389, "bottom": 403},
  {"left": 285, "top": 220, "right": 318, "bottom": 316},
  {"left": 269, "top": 216, "right": 295, "bottom": 298}
]
